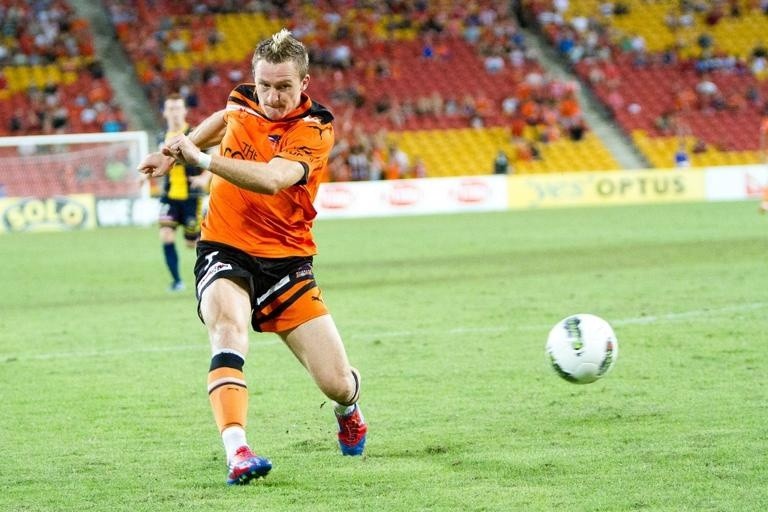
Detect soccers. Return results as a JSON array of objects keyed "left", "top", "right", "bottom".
[{"left": 546, "top": 314, "right": 619, "bottom": 385}]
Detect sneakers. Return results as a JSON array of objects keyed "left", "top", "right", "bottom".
[
  {"left": 165, "top": 279, "right": 186, "bottom": 292},
  {"left": 226, "top": 444, "right": 273, "bottom": 486},
  {"left": 331, "top": 398, "right": 369, "bottom": 457}
]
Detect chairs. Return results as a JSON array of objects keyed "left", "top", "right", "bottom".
[{"left": 0, "top": 0, "right": 768, "bottom": 196}]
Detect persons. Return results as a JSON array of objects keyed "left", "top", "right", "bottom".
[
  {"left": 157, "top": 92, "right": 215, "bottom": 295},
  {"left": 137, "top": 26, "right": 370, "bottom": 487},
  {"left": 1, "top": 0, "right": 768, "bottom": 191}
]
